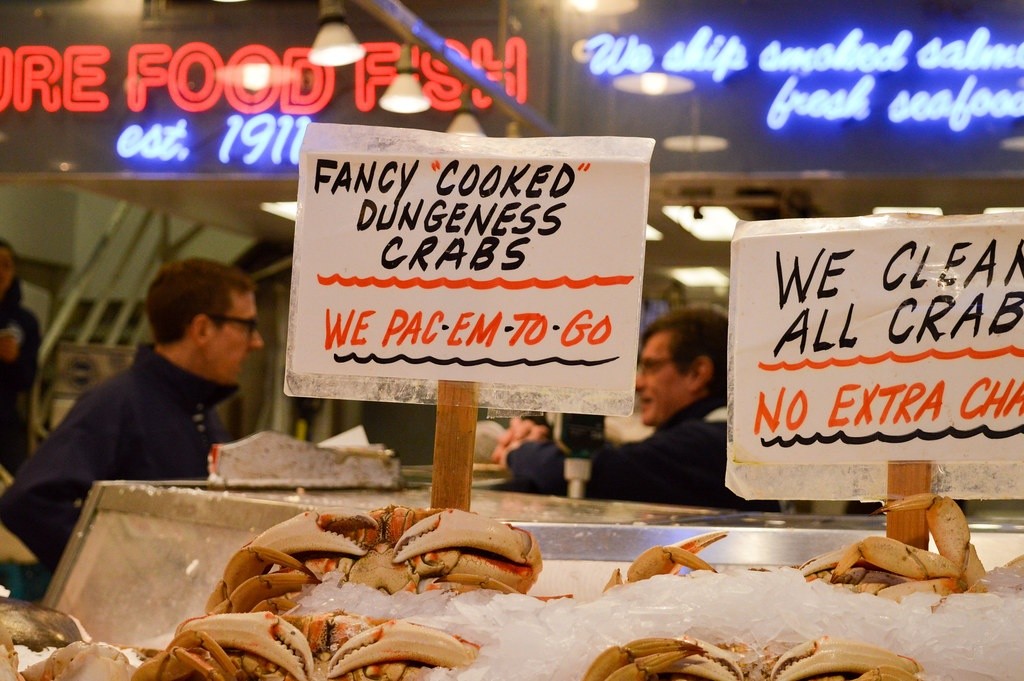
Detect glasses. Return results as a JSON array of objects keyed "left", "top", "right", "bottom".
[
  {"left": 188, "top": 313, "right": 257, "bottom": 335},
  {"left": 637, "top": 357, "right": 677, "bottom": 377}
]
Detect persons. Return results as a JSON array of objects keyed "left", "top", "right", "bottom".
[
  {"left": 492, "top": 307, "right": 790, "bottom": 512},
  {"left": 0, "top": 237, "right": 40, "bottom": 476},
  {"left": 0, "top": 257, "right": 266, "bottom": 579}
]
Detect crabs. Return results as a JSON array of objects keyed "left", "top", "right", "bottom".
[
  {"left": 0, "top": 544, "right": 322, "bottom": 680},
  {"left": 240, "top": 504, "right": 575, "bottom": 601},
  {"left": 582, "top": 633, "right": 926, "bottom": 681},
  {"left": 601, "top": 493, "right": 988, "bottom": 598},
  {"left": 126, "top": 609, "right": 487, "bottom": 681}
]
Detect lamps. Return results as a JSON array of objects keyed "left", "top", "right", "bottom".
[
  {"left": 308, "top": 0, "right": 368, "bottom": 66},
  {"left": 444, "top": 91, "right": 489, "bottom": 137},
  {"left": 611, "top": 57, "right": 695, "bottom": 97},
  {"left": 376, "top": 43, "right": 432, "bottom": 114},
  {"left": 566, "top": 0, "right": 640, "bottom": 19}
]
